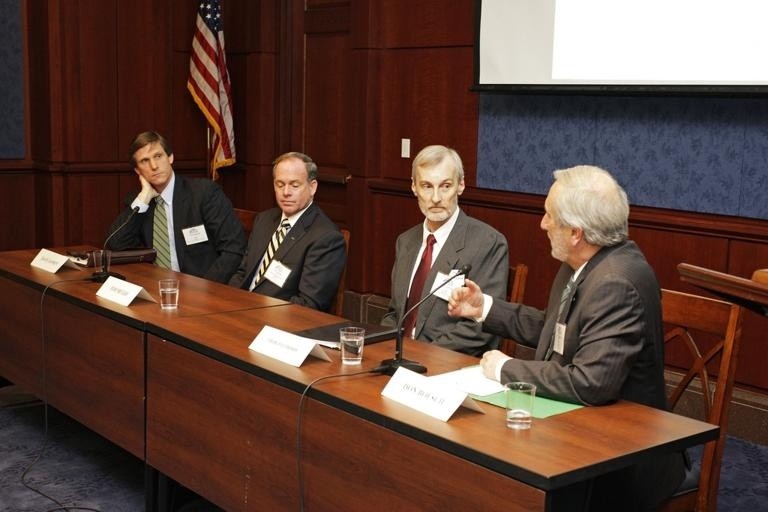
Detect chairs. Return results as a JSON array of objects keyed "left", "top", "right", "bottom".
[
  {"left": 500, "top": 262, "right": 529, "bottom": 355},
  {"left": 607, "top": 288, "right": 741, "bottom": 508},
  {"left": 323, "top": 230, "right": 349, "bottom": 314},
  {"left": 233, "top": 211, "right": 256, "bottom": 236}
]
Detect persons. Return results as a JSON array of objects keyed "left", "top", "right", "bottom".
[
  {"left": 446, "top": 165, "right": 695, "bottom": 512},
  {"left": 105, "top": 130, "right": 245, "bottom": 285},
  {"left": 227, "top": 152, "right": 347, "bottom": 313},
  {"left": 379, "top": 144, "right": 508, "bottom": 358}
]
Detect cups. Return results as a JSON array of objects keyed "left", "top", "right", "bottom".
[
  {"left": 159, "top": 278, "right": 180, "bottom": 311},
  {"left": 340, "top": 327, "right": 365, "bottom": 365},
  {"left": 504, "top": 380, "right": 537, "bottom": 430},
  {"left": 93, "top": 249, "right": 112, "bottom": 274}
]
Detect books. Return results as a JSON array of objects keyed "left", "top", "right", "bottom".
[{"left": 294, "top": 321, "right": 405, "bottom": 351}]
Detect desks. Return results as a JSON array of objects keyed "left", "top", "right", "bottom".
[
  {"left": 146, "top": 303, "right": 719, "bottom": 512},
  {"left": 1, "top": 242, "right": 293, "bottom": 507}
]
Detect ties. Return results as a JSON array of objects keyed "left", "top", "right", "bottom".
[
  {"left": 152, "top": 196, "right": 171, "bottom": 270},
  {"left": 544, "top": 276, "right": 574, "bottom": 361},
  {"left": 255, "top": 218, "right": 291, "bottom": 286},
  {"left": 403, "top": 235, "right": 437, "bottom": 338}
]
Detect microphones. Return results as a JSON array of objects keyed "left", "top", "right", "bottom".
[
  {"left": 380, "top": 263, "right": 472, "bottom": 377},
  {"left": 91, "top": 206, "right": 140, "bottom": 283}
]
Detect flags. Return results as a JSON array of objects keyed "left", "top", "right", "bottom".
[{"left": 185, "top": 0, "right": 236, "bottom": 182}]
